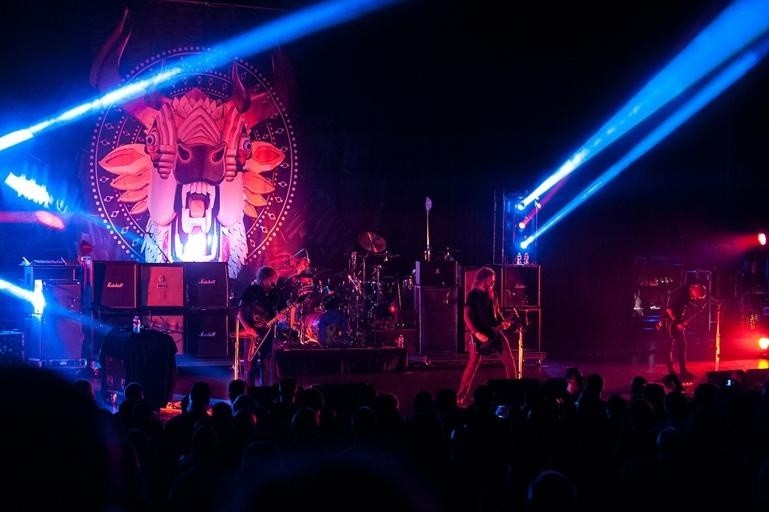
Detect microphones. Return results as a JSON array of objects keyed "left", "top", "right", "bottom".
[
  {"left": 271, "top": 282, "right": 281, "bottom": 293},
  {"left": 505, "top": 287, "right": 520, "bottom": 297}
]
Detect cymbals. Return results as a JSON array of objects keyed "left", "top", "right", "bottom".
[{"left": 358, "top": 231, "right": 387, "bottom": 252}]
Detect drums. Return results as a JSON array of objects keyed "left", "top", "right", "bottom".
[
  {"left": 304, "top": 309, "right": 346, "bottom": 346},
  {"left": 297, "top": 276, "right": 318, "bottom": 298},
  {"left": 321, "top": 285, "right": 337, "bottom": 301}
]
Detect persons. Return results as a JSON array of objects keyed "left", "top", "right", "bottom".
[
  {"left": 2, "top": 365, "right": 769, "bottom": 512},
  {"left": 663, "top": 279, "right": 709, "bottom": 387},
  {"left": 454, "top": 263, "right": 522, "bottom": 411},
  {"left": 237, "top": 265, "right": 290, "bottom": 388}
]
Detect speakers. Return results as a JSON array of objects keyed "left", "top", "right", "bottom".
[
  {"left": 91, "top": 259, "right": 137, "bottom": 310},
  {"left": 184, "top": 310, "right": 229, "bottom": 357},
  {"left": 413, "top": 285, "right": 458, "bottom": 354},
  {"left": 184, "top": 261, "right": 230, "bottom": 311},
  {"left": 500, "top": 309, "right": 542, "bottom": 353},
  {"left": 494, "top": 263, "right": 541, "bottom": 309},
  {"left": 91, "top": 310, "right": 140, "bottom": 360},
  {"left": 138, "top": 262, "right": 184, "bottom": 310},
  {"left": 34, "top": 278, "right": 84, "bottom": 314},
  {"left": 29, "top": 313, "right": 84, "bottom": 360},
  {"left": 415, "top": 261, "right": 458, "bottom": 287},
  {"left": 140, "top": 310, "right": 184, "bottom": 357}
]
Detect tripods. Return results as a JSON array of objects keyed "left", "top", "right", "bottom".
[
  {"left": 347, "top": 296, "right": 364, "bottom": 348},
  {"left": 294, "top": 315, "right": 319, "bottom": 351},
  {"left": 280, "top": 302, "right": 301, "bottom": 350}
]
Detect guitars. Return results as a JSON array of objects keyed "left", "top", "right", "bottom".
[
  {"left": 229, "top": 302, "right": 296, "bottom": 362},
  {"left": 471, "top": 297, "right": 528, "bottom": 355}
]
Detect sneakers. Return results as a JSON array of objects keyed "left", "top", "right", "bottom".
[
  {"left": 454, "top": 394, "right": 466, "bottom": 406},
  {"left": 666, "top": 371, "right": 696, "bottom": 382}
]
